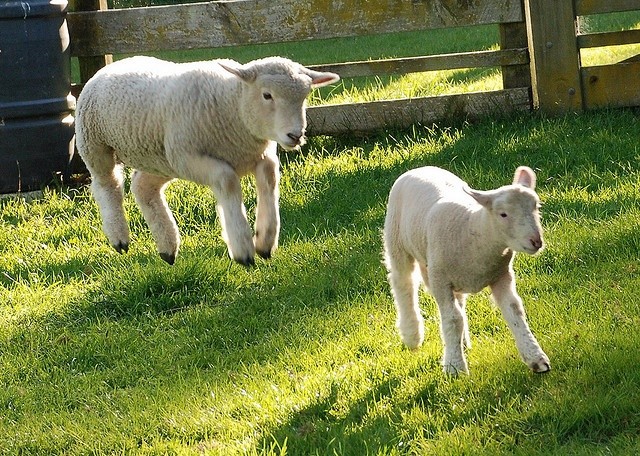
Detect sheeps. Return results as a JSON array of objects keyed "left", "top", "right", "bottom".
[
  {"left": 74, "top": 55, "right": 341, "bottom": 267},
  {"left": 379, "top": 165, "right": 553, "bottom": 378}
]
